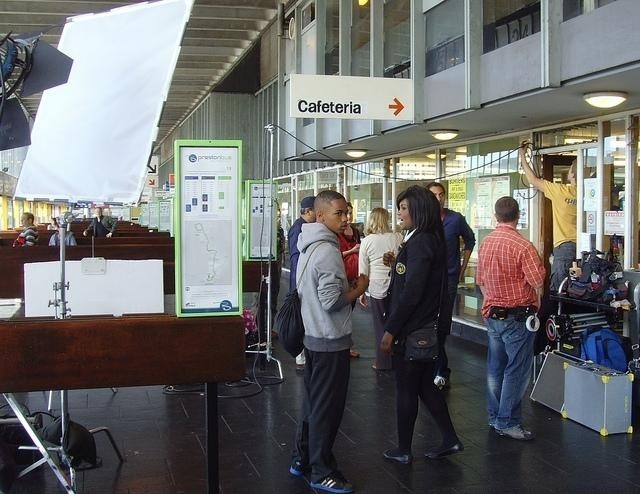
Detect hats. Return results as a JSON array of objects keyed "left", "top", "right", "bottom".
[{"left": 301, "top": 196, "right": 316, "bottom": 208}]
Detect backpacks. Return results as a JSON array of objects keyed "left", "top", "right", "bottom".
[
  {"left": 579, "top": 325, "right": 628, "bottom": 373},
  {"left": 275, "top": 287, "right": 307, "bottom": 359}
]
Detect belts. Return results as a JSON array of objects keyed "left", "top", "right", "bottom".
[{"left": 492, "top": 307, "right": 534, "bottom": 315}]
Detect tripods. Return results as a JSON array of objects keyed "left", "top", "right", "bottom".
[
  {"left": 245, "top": 134, "right": 283, "bottom": 379},
  {"left": 18, "top": 227, "right": 124, "bottom": 494}
]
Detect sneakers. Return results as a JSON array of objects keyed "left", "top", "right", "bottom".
[
  {"left": 495, "top": 424, "right": 536, "bottom": 441},
  {"left": 309, "top": 472, "right": 354, "bottom": 494},
  {"left": 289, "top": 462, "right": 306, "bottom": 476},
  {"left": 349, "top": 351, "right": 361, "bottom": 359}
]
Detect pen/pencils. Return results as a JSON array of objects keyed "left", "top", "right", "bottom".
[{"left": 0, "top": 304, "right": 16, "bottom": 306}]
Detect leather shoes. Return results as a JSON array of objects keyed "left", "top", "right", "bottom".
[
  {"left": 424, "top": 438, "right": 464, "bottom": 459},
  {"left": 382, "top": 448, "right": 413, "bottom": 465}
]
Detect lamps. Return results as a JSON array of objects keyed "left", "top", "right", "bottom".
[
  {"left": 344, "top": 148, "right": 368, "bottom": 159},
  {"left": 427, "top": 129, "right": 460, "bottom": 143},
  {"left": 583, "top": 90, "right": 630, "bottom": 110}
]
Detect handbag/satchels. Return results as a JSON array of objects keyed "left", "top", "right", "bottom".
[{"left": 401, "top": 323, "right": 442, "bottom": 363}]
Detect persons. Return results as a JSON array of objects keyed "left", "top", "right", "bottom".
[
  {"left": 255, "top": 226, "right": 285, "bottom": 339},
  {"left": 46, "top": 217, "right": 60, "bottom": 231},
  {"left": 475, "top": 195, "right": 548, "bottom": 441},
  {"left": 423, "top": 182, "right": 476, "bottom": 391},
  {"left": 82, "top": 207, "right": 119, "bottom": 237},
  {"left": 48, "top": 215, "right": 78, "bottom": 245},
  {"left": 338, "top": 201, "right": 362, "bottom": 357},
  {"left": 381, "top": 185, "right": 463, "bottom": 465},
  {"left": 357, "top": 207, "right": 401, "bottom": 374},
  {"left": 12, "top": 211, "right": 41, "bottom": 247},
  {"left": 288, "top": 190, "right": 370, "bottom": 493},
  {"left": 518, "top": 138, "right": 578, "bottom": 290},
  {"left": 289, "top": 196, "right": 317, "bottom": 373}
]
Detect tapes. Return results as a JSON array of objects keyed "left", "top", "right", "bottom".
[{"left": 526, "top": 315, "right": 541, "bottom": 332}]
